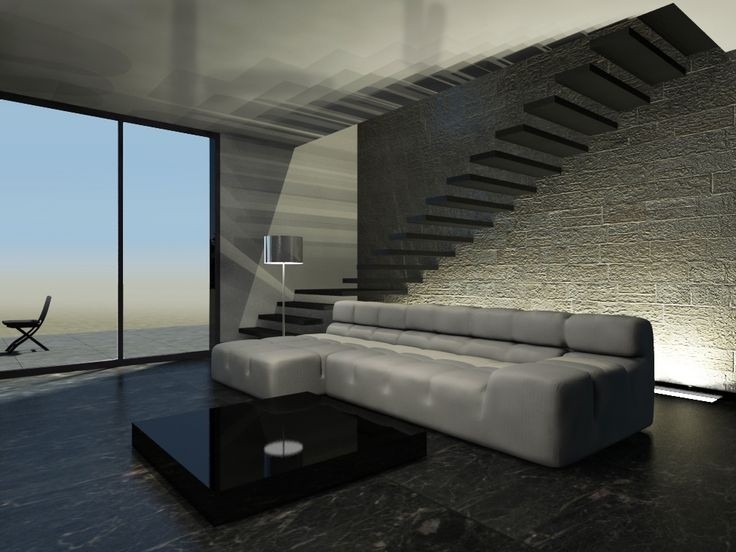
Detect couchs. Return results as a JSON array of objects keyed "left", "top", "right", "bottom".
[{"left": 211, "top": 300, "right": 656, "bottom": 467}]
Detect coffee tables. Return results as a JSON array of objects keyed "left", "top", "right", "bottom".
[{"left": 132, "top": 390, "right": 429, "bottom": 525}]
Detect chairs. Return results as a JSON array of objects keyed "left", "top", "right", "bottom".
[{"left": 1, "top": 296, "right": 51, "bottom": 354}]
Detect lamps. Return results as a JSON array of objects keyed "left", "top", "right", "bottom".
[{"left": 263, "top": 235, "right": 303, "bottom": 337}]
